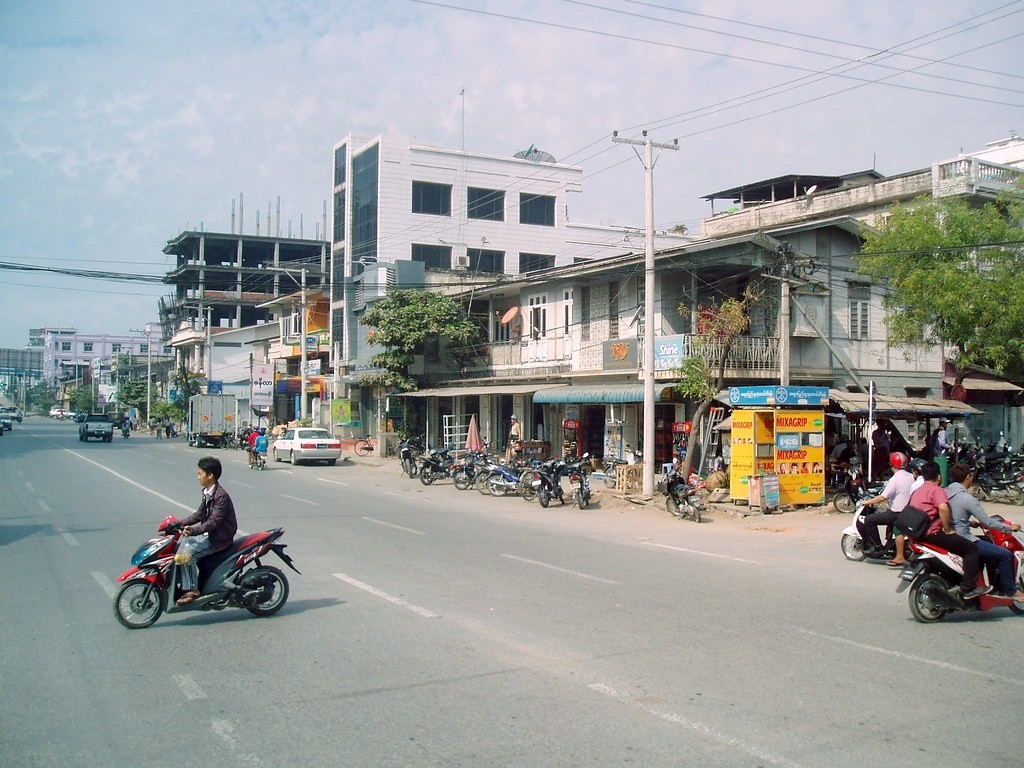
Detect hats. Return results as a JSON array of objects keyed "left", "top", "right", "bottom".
[{"left": 939, "top": 417, "right": 950, "bottom": 422}]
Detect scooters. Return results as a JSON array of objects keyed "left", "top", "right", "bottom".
[
  {"left": 841, "top": 482, "right": 913, "bottom": 563},
  {"left": 894, "top": 515, "right": 1024, "bottom": 625},
  {"left": 246, "top": 444, "right": 268, "bottom": 470},
  {"left": 122, "top": 428, "right": 133, "bottom": 439},
  {"left": 947, "top": 438, "right": 1023, "bottom": 504},
  {"left": 833, "top": 456, "right": 890, "bottom": 514},
  {"left": 111, "top": 515, "right": 305, "bottom": 629},
  {"left": 219, "top": 428, "right": 239, "bottom": 450}
]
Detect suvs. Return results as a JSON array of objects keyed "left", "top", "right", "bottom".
[{"left": 49, "top": 409, "right": 77, "bottom": 420}]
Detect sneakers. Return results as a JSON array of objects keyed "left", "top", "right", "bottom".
[{"left": 963, "top": 585, "right": 993, "bottom": 599}]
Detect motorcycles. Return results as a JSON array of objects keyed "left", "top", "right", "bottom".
[
  {"left": 395, "top": 432, "right": 571, "bottom": 508},
  {"left": 563, "top": 452, "right": 593, "bottom": 509},
  {"left": 658, "top": 464, "right": 723, "bottom": 529}
]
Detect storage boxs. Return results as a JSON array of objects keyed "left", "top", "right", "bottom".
[{"left": 590, "top": 459, "right": 602, "bottom": 469}]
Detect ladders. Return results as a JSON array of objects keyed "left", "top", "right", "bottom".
[{"left": 696, "top": 407, "right": 724, "bottom": 489}]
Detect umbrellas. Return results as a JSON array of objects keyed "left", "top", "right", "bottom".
[{"left": 465, "top": 415, "right": 482, "bottom": 450}]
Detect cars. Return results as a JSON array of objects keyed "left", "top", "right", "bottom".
[
  {"left": 0, "top": 406, "right": 22, "bottom": 435},
  {"left": 272, "top": 428, "right": 342, "bottom": 467}
]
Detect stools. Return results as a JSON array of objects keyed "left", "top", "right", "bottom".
[{"left": 661, "top": 463, "right": 672, "bottom": 479}]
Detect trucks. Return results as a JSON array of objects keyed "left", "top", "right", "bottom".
[{"left": 186, "top": 392, "right": 237, "bottom": 448}]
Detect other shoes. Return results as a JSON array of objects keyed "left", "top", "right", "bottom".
[{"left": 862, "top": 544, "right": 884, "bottom": 555}]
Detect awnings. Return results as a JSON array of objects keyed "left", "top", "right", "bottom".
[
  {"left": 532, "top": 383, "right": 680, "bottom": 403},
  {"left": 394, "top": 383, "right": 568, "bottom": 397},
  {"left": 944, "top": 377, "right": 1024, "bottom": 397},
  {"left": 828, "top": 391, "right": 987, "bottom": 416}
]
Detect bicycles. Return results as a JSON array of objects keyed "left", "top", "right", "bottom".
[{"left": 354, "top": 434, "right": 376, "bottom": 457}]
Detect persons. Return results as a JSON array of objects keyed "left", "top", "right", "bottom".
[
  {"left": 177, "top": 457, "right": 237, "bottom": 604},
  {"left": 133, "top": 415, "right": 138, "bottom": 432},
  {"left": 886, "top": 458, "right": 928, "bottom": 567},
  {"left": 506, "top": 414, "right": 521, "bottom": 463},
  {"left": 909, "top": 462, "right": 993, "bottom": 600},
  {"left": 148, "top": 416, "right": 171, "bottom": 440},
  {"left": 831, "top": 434, "right": 868, "bottom": 464},
  {"left": 861, "top": 451, "right": 916, "bottom": 560},
  {"left": 246, "top": 426, "right": 269, "bottom": 463},
  {"left": 872, "top": 419, "right": 905, "bottom": 481},
  {"left": 930, "top": 417, "right": 952, "bottom": 456},
  {"left": 17, "top": 412, "right": 22, "bottom": 416},
  {"left": 122, "top": 417, "right": 133, "bottom": 433},
  {"left": 945, "top": 462, "right": 1024, "bottom": 603},
  {"left": 61, "top": 410, "right": 63, "bottom": 415}
]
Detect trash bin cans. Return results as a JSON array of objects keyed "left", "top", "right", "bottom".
[
  {"left": 347, "top": 421, "right": 362, "bottom": 439},
  {"left": 334, "top": 423, "right": 351, "bottom": 440}
]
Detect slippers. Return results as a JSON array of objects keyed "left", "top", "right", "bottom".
[
  {"left": 176, "top": 591, "right": 200, "bottom": 605},
  {"left": 1005, "top": 592, "right": 1024, "bottom": 603},
  {"left": 886, "top": 560, "right": 904, "bottom": 566}
]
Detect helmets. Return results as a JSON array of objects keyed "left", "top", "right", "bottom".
[
  {"left": 890, "top": 452, "right": 907, "bottom": 469},
  {"left": 253, "top": 426, "right": 259, "bottom": 431},
  {"left": 258, "top": 429, "right": 265, "bottom": 434},
  {"left": 430, "top": 449, "right": 437, "bottom": 455},
  {"left": 510, "top": 415, "right": 518, "bottom": 419},
  {"left": 908, "top": 457, "right": 928, "bottom": 473}
]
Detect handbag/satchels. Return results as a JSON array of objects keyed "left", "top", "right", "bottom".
[
  {"left": 894, "top": 505, "right": 931, "bottom": 541},
  {"left": 174, "top": 536, "right": 193, "bottom": 565}
]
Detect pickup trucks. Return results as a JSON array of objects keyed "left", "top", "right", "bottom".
[{"left": 79, "top": 413, "right": 115, "bottom": 442}]
